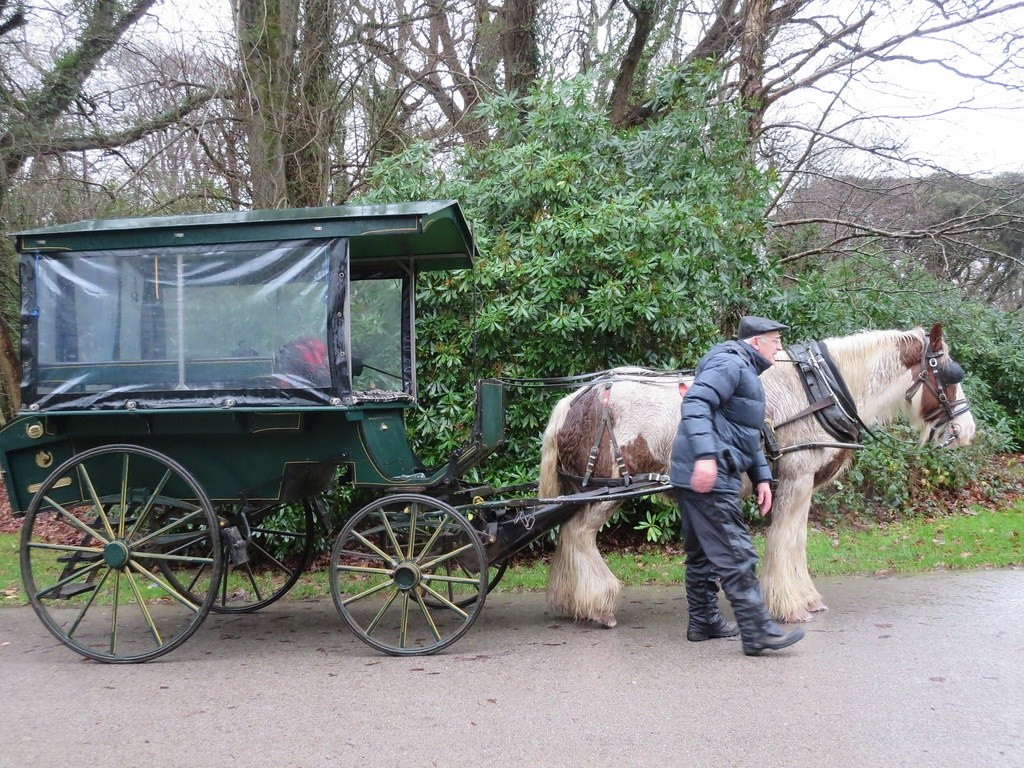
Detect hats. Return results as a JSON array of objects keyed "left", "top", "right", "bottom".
[{"left": 739, "top": 316, "right": 789, "bottom": 338}]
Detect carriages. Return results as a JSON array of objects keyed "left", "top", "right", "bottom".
[{"left": 1, "top": 199, "right": 977, "bottom": 665}]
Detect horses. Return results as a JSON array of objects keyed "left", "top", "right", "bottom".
[{"left": 538, "top": 322, "right": 975, "bottom": 628}]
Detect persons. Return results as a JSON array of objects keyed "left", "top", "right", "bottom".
[{"left": 669, "top": 315, "right": 805, "bottom": 655}]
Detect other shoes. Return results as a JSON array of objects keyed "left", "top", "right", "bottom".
[
  {"left": 744, "top": 628, "right": 804, "bottom": 655},
  {"left": 687, "top": 621, "right": 739, "bottom": 642}
]
